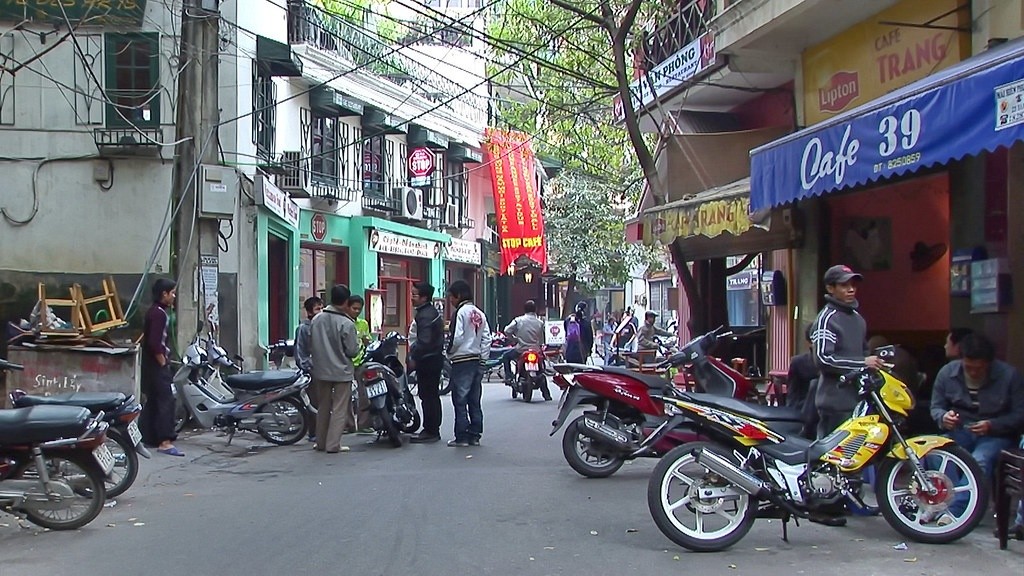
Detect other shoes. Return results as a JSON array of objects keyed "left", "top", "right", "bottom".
[
  {"left": 937, "top": 514, "right": 952, "bottom": 525},
  {"left": 502, "top": 379, "right": 509, "bottom": 383},
  {"left": 808, "top": 512, "right": 847, "bottom": 526},
  {"left": 326, "top": 446, "right": 350, "bottom": 454},
  {"left": 924, "top": 511, "right": 937, "bottom": 521},
  {"left": 858, "top": 507, "right": 880, "bottom": 517},
  {"left": 313, "top": 444, "right": 326, "bottom": 451},
  {"left": 545, "top": 397, "right": 552, "bottom": 401},
  {"left": 358, "top": 428, "right": 374, "bottom": 433},
  {"left": 342, "top": 429, "right": 356, "bottom": 434}
]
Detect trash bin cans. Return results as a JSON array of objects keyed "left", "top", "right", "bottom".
[{"left": 5, "top": 343, "right": 143, "bottom": 416}]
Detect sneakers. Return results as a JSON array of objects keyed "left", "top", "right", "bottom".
[
  {"left": 410, "top": 429, "right": 441, "bottom": 442},
  {"left": 993, "top": 523, "right": 1024, "bottom": 540},
  {"left": 447, "top": 441, "right": 480, "bottom": 446}
]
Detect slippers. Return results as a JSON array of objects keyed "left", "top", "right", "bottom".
[{"left": 157, "top": 446, "right": 185, "bottom": 456}]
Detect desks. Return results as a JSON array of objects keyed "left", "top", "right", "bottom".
[{"left": 744, "top": 377, "right": 773, "bottom": 405}]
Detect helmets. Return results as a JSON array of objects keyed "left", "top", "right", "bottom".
[{"left": 524, "top": 300, "right": 536, "bottom": 312}]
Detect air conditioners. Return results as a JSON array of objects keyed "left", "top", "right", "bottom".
[
  {"left": 281, "top": 151, "right": 311, "bottom": 198},
  {"left": 439, "top": 203, "right": 458, "bottom": 229},
  {"left": 392, "top": 187, "right": 423, "bottom": 223}
]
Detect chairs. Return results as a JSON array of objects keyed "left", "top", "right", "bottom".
[
  {"left": 683, "top": 372, "right": 697, "bottom": 393},
  {"left": 731, "top": 358, "right": 747, "bottom": 376},
  {"left": 996, "top": 449, "right": 1024, "bottom": 550}
]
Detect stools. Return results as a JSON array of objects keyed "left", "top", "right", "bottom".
[
  {"left": 69, "top": 276, "right": 127, "bottom": 336},
  {"left": 37, "top": 282, "right": 81, "bottom": 336}
]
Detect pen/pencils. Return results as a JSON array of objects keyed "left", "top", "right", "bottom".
[{"left": 956, "top": 413, "right": 960, "bottom": 417}]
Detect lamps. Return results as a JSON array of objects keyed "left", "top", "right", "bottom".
[{"left": 142, "top": 104, "right": 151, "bottom": 121}]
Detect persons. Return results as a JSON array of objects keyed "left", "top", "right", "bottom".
[
  {"left": 592, "top": 309, "right": 601, "bottom": 324},
  {"left": 294, "top": 297, "right": 324, "bottom": 441},
  {"left": 342, "top": 295, "right": 374, "bottom": 434},
  {"left": 446, "top": 280, "right": 492, "bottom": 447},
  {"left": 138, "top": 279, "right": 186, "bottom": 456},
  {"left": 603, "top": 318, "right": 615, "bottom": 367},
  {"left": 637, "top": 311, "right": 678, "bottom": 363},
  {"left": 866, "top": 336, "right": 918, "bottom": 397},
  {"left": 786, "top": 322, "right": 818, "bottom": 414},
  {"left": 310, "top": 284, "right": 359, "bottom": 453},
  {"left": 810, "top": 264, "right": 886, "bottom": 525},
  {"left": 409, "top": 281, "right": 446, "bottom": 443},
  {"left": 920, "top": 327, "right": 1024, "bottom": 540},
  {"left": 564, "top": 301, "right": 593, "bottom": 383},
  {"left": 503, "top": 300, "right": 552, "bottom": 401}
]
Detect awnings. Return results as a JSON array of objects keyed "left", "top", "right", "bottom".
[
  {"left": 748, "top": 35, "right": 1024, "bottom": 215},
  {"left": 642, "top": 175, "right": 771, "bottom": 246}
]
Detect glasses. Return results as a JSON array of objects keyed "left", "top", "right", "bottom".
[{"left": 411, "top": 293, "right": 419, "bottom": 297}]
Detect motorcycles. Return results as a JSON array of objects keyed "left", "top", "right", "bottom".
[
  {"left": 0, "top": 358, "right": 152, "bottom": 531},
  {"left": 548, "top": 324, "right": 760, "bottom": 482},
  {"left": 631, "top": 343, "right": 989, "bottom": 552},
  {"left": 170, "top": 319, "right": 317, "bottom": 446},
  {"left": 357, "top": 330, "right": 421, "bottom": 449},
  {"left": 504, "top": 331, "right": 543, "bottom": 402},
  {"left": 613, "top": 331, "right": 680, "bottom": 373},
  {"left": 486, "top": 346, "right": 519, "bottom": 382},
  {"left": 406, "top": 349, "right": 456, "bottom": 395}
]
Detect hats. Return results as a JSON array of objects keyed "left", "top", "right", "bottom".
[
  {"left": 646, "top": 310, "right": 659, "bottom": 316},
  {"left": 825, "top": 266, "right": 864, "bottom": 283}
]
